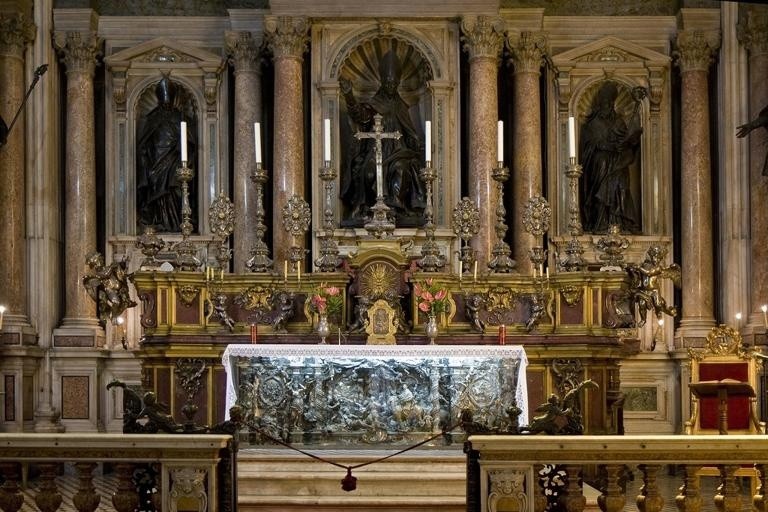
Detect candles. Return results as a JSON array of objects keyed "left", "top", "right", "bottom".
[
  {"left": 254, "top": 119, "right": 262, "bottom": 164},
  {"left": 283, "top": 258, "right": 301, "bottom": 283},
  {"left": 323, "top": 117, "right": 332, "bottom": 162},
  {"left": 496, "top": 119, "right": 505, "bottom": 161},
  {"left": 566, "top": 116, "right": 577, "bottom": 160},
  {"left": 531, "top": 262, "right": 549, "bottom": 280},
  {"left": 206, "top": 264, "right": 226, "bottom": 283},
  {"left": 179, "top": 119, "right": 191, "bottom": 166},
  {"left": 458, "top": 259, "right": 477, "bottom": 282},
  {"left": 424, "top": 120, "right": 433, "bottom": 162}
]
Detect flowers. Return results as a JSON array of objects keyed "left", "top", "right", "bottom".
[
  {"left": 304, "top": 282, "right": 343, "bottom": 315},
  {"left": 415, "top": 278, "right": 448, "bottom": 317}
]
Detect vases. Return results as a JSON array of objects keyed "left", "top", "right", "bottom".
[
  {"left": 316, "top": 315, "right": 329, "bottom": 344},
  {"left": 426, "top": 312, "right": 440, "bottom": 344}
]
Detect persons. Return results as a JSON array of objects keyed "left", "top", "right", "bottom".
[
  {"left": 140, "top": 75, "right": 195, "bottom": 230},
  {"left": 581, "top": 81, "right": 642, "bottom": 232},
  {"left": 339, "top": 52, "right": 432, "bottom": 228},
  {"left": 734, "top": 100, "right": 764, "bottom": 180}
]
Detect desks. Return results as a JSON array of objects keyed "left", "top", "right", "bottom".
[{"left": 222, "top": 343, "right": 525, "bottom": 450}]
